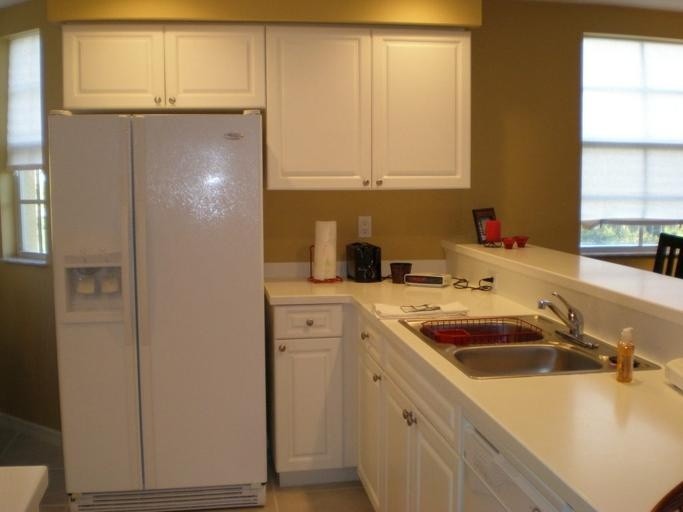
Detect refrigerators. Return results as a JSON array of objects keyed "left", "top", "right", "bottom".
[{"left": 45, "top": 116, "right": 265, "bottom": 512}]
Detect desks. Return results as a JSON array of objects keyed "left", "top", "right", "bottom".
[{"left": 1, "top": 465, "right": 48, "bottom": 510}]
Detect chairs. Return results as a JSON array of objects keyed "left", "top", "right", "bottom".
[{"left": 652, "top": 229, "right": 683, "bottom": 280}]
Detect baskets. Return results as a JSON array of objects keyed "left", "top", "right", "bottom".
[{"left": 418, "top": 315, "right": 544, "bottom": 347}]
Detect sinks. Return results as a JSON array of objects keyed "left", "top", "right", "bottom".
[
  {"left": 398, "top": 316, "right": 544, "bottom": 344},
  {"left": 453, "top": 345, "right": 603, "bottom": 381}
]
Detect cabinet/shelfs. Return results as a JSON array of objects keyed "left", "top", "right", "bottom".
[
  {"left": 381, "top": 371, "right": 460, "bottom": 511},
  {"left": 460, "top": 454, "right": 510, "bottom": 511},
  {"left": 460, "top": 390, "right": 600, "bottom": 511},
  {"left": 358, "top": 303, "right": 380, "bottom": 371},
  {"left": 62, "top": 20, "right": 264, "bottom": 111},
  {"left": 359, "top": 344, "right": 380, "bottom": 510},
  {"left": 382, "top": 321, "right": 460, "bottom": 450},
  {"left": 265, "top": 24, "right": 470, "bottom": 188},
  {"left": 272, "top": 305, "right": 357, "bottom": 339},
  {"left": 272, "top": 338, "right": 358, "bottom": 486}
]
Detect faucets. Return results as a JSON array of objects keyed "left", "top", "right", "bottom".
[{"left": 537, "top": 292, "right": 584, "bottom": 338}]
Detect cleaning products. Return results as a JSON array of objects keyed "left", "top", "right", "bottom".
[{"left": 616, "top": 327, "right": 635, "bottom": 382}]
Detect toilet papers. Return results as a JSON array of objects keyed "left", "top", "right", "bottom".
[{"left": 312, "top": 220, "right": 338, "bottom": 280}]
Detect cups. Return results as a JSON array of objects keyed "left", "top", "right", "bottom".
[
  {"left": 390, "top": 262, "right": 411, "bottom": 283},
  {"left": 501, "top": 234, "right": 533, "bottom": 249}
]
